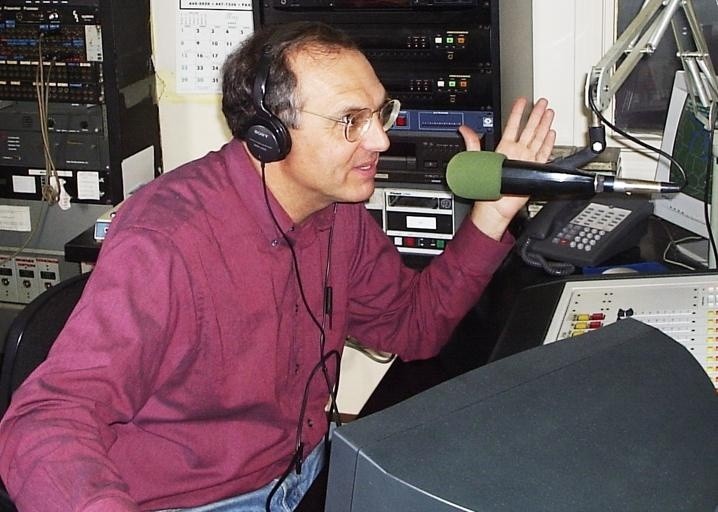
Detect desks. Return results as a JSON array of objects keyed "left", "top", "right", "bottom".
[{"left": 291, "top": 191, "right": 718, "bottom": 512}]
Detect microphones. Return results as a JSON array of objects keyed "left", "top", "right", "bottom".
[{"left": 446, "top": 150, "right": 680, "bottom": 201}]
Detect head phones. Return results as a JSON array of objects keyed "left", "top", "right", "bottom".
[{"left": 245, "top": 22, "right": 323, "bottom": 162}]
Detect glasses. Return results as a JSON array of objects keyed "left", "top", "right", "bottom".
[{"left": 295, "top": 99, "right": 401, "bottom": 142}]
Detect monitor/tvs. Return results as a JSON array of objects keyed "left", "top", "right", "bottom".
[
  {"left": 323, "top": 317, "right": 718, "bottom": 511},
  {"left": 650, "top": 70, "right": 718, "bottom": 269}
]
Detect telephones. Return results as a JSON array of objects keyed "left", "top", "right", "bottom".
[{"left": 514, "top": 193, "right": 653, "bottom": 266}]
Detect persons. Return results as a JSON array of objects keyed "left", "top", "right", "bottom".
[{"left": 0, "top": 21, "right": 557, "bottom": 512}]
